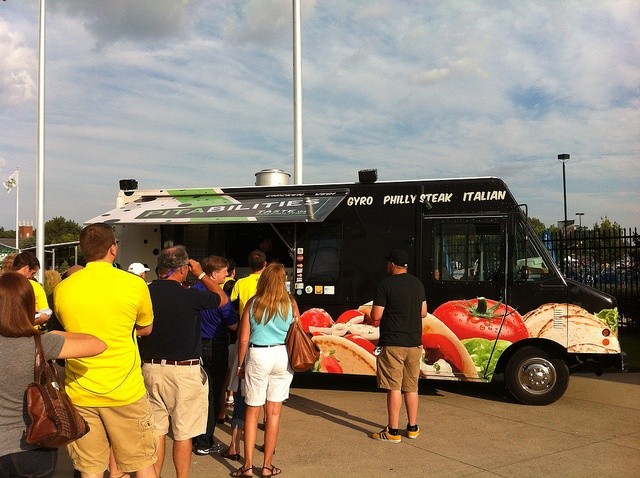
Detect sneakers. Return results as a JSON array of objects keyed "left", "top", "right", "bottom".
[
  {"left": 370, "top": 425, "right": 401, "bottom": 443},
  {"left": 224, "top": 398, "right": 235, "bottom": 408},
  {"left": 196, "top": 442, "right": 226, "bottom": 456},
  {"left": 407, "top": 426, "right": 420, "bottom": 439}
]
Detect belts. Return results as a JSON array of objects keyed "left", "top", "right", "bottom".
[
  {"left": 143, "top": 359, "right": 199, "bottom": 366},
  {"left": 249, "top": 343, "right": 286, "bottom": 348}
]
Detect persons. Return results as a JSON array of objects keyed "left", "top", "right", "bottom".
[
  {"left": 141, "top": 244, "right": 228, "bottom": 478},
  {"left": 371, "top": 250, "right": 427, "bottom": 443},
  {"left": 220, "top": 251, "right": 275, "bottom": 461},
  {"left": 52, "top": 222, "right": 159, "bottom": 478},
  {"left": 128, "top": 262, "right": 150, "bottom": 283},
  {"left": 0, "top": 272, "right": 108, "bottom": 478},
  {"left": 229, "top": 262, "right": 300, "bottom": 477},
  {"left": 13, "top": 251, "right": 53, "bottom": 331},
  {"left": 217, "top": 259, "right": 239, "bottom": 425},
  {"left": 190, "top": 255, "right": 239, "bottom": 456},
  {"left": 33, "top": 269, "right": 66, "bottom": 367}
]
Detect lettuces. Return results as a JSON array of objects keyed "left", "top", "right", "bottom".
[{"left": 595, "top": 307, "right": 619, "bottom": 337}]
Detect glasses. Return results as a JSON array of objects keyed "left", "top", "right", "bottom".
[
  {"left": 107, "top": 240, "right": 119, "bottom": 250},
  {"left": 158, "top": 264, "right": 191, "bottom": 279}
]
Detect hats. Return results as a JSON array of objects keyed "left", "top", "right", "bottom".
[
  {"left": 384, "top": 249, "right": 410, "bottom": 266},
  {"left": 128, "top": 262, "right": 151, "bottom": 276}
]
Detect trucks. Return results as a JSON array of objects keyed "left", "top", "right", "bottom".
[{"left": 84, "top": 169, "right": 622, "bottom": 406}]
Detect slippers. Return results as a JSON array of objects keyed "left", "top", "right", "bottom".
[
  {"left": 219, "top": 450, "right": 240, "bottom": 461},
  {"left": 262, "top": 444, "right": 275, "bottom": 455}
]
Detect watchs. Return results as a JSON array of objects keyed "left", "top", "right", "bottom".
[
  {"left": 237, "top": 366, "right": 242, "bottom": 369},
  {"left": 198, "top": 271, "right": 206, "bottom": 280}
]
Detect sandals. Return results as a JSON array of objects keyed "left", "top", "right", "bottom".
[
  {"left": 230, "top": 465, "right": 254, "bottom": 478},
  {"left": 262, "top": 465, "right": 281, "bottom": 478}
]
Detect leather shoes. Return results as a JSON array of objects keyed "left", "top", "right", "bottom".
[{"left": 216, "top": 414, "right": 231, "bottom": 425}]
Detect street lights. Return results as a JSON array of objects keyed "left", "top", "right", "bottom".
[{"left": 555, "top": 152, "right": 571, "bottom": 235}]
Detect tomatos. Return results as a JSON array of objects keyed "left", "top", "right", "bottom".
[
  {"left": 296, "top": 306, "right": 335, "bottom": 335},
  {"left": 432, "top": 296, "right": 529, "bottom": 344}
]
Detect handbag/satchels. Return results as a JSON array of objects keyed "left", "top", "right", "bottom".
[
  {"left": 285, "top": 296, "right": 320, "bottom": 373},
  {"left": 23, "top": 333, "right": 90, "bottom": 449}
]
